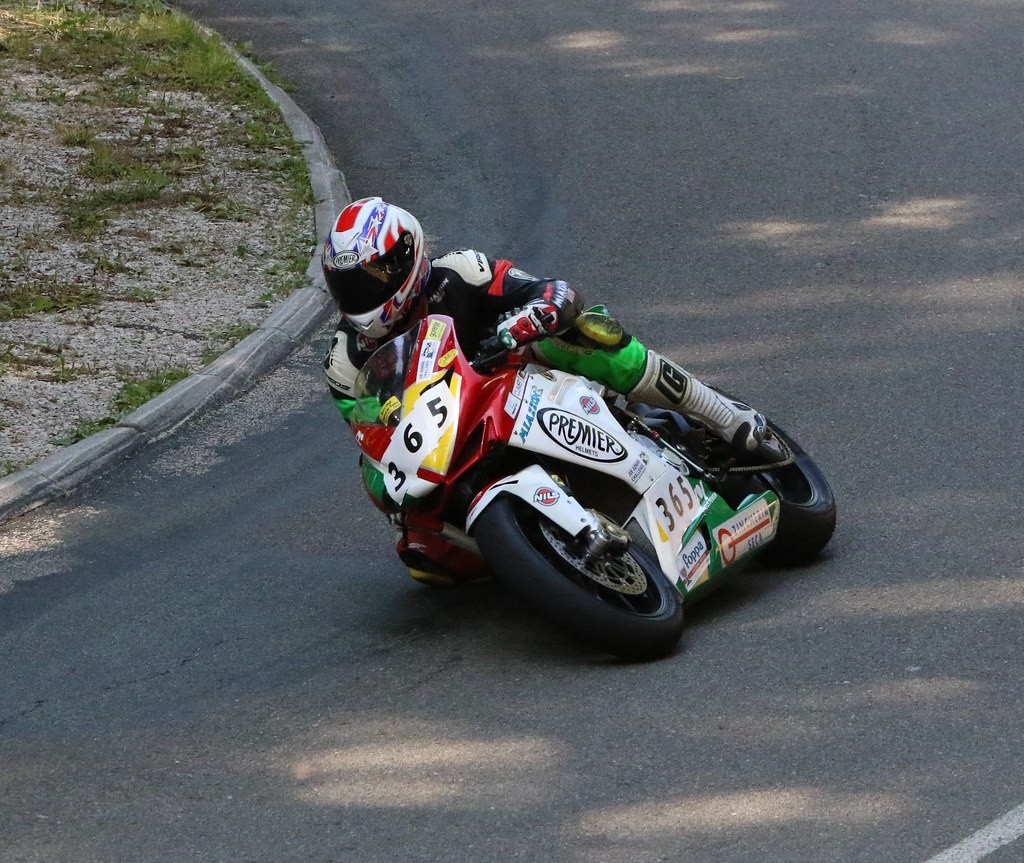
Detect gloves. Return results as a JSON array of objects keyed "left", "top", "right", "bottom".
[{"left": 491, "top": 297, "right": 560, "bottom": 350}]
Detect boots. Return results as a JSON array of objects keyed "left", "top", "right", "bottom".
[{"left": 624, "top": 350, "right": 767, "bottom": 451}]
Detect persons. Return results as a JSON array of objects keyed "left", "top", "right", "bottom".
[{"left": 321, "top": 197, "right": 768, "bottom": 587}]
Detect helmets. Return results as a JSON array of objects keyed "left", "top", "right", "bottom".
[{"left": 321, "top": 196, "right": 432, "bottom": 339}]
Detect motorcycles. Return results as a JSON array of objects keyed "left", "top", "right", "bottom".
[{"left": 351, "top": 291, "right": 836, "bottom": 665}]
